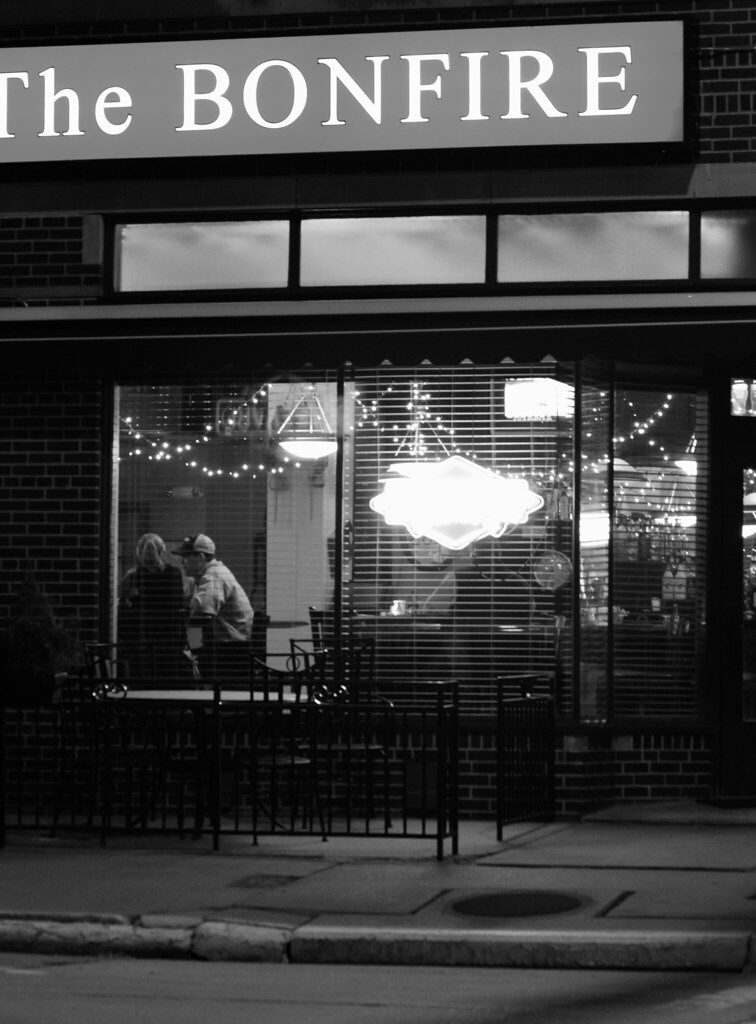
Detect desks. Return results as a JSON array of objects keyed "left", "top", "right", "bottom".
[{"left": 104, "top": 687, "right": 311, "bottom": 833}]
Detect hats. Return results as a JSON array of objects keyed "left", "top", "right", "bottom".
[{"left": 172, "top": 532, "right": 216, "bottom": 555}]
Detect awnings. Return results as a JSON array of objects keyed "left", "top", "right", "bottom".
[{"left": 0, "top": 291, "right": 756, "bottom": 389}]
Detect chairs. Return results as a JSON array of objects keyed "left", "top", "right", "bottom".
[{"left": 70, "top": 633, "right": 393, "bottom": 844}]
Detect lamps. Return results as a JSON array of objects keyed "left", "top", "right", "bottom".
[{"left": 276, "top": 381, "right": 339, "bottom": 460}]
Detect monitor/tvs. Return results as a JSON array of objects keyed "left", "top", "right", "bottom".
[{"left": 611, "top": 561, "right": 662, "bottom": 607}]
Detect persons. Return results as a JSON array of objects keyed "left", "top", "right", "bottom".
[
  {"left": 119, "top": 533, "right": 202, "bottom": 691},
  {"left": 172, "top": 534, "right": 254, "bottom": 691},
  {"left": 426, "top": 537, "right": 536, "bottom": 625}
]
